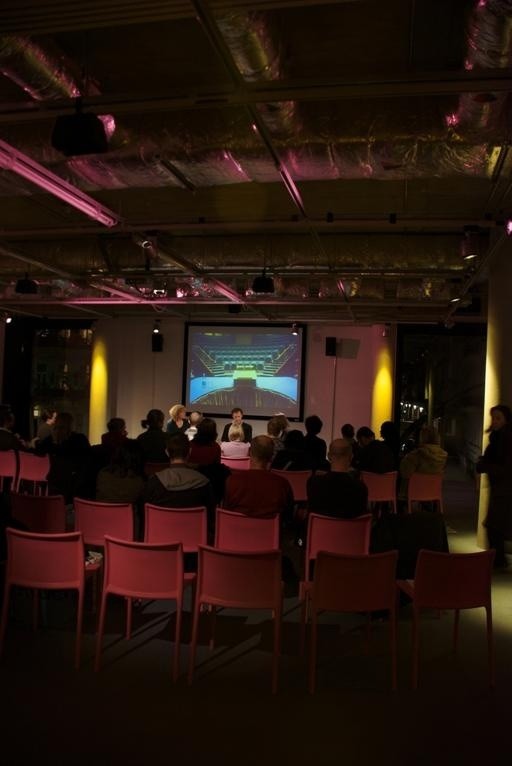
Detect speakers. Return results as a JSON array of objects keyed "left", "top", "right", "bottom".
[
  {"left": 151, "top": 333, "right": 163, "bottom": 352},
  {"left": 326, "top": 337, "right": 336, "bottom": 356}
]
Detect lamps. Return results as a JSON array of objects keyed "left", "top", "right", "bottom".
[
  {"left": 13, "top": 271, "right": 41, "bottom": 295},
  {"left": 151, "top": 319, "right": 161, "bottom": 334},
  {"left": 289, "top": 321, "right": 300, "bottom": 335},
  {"left": 250, "top": 253, "right": 275, "bottom": 294}
]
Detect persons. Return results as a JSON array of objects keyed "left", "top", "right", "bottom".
[
  {"left": 224, "top": 408, "right": 252, "bottom": 443},
  {"left": 268, "top": 415, "right": 398, "bottom": 474},
  {"left": 224, "top": 435, "right": 297, "bottom": 554},
  {"left": 147, "top": 433, "right": 218, "bottom": 572},
  {"left": 2, "top": 404, "right": 222, "bottom": 472},
  {"left": 305, "top": 439, "right": 368, "bottom": 521},
  {"left": 465, "top": 404, "right": 512, "bottom": 571},
  {"left": 96, "top": 441, "right": 151, "bottom": 543},
  {"left": 398, "top": 426, "right": 449, "bottom": 514}
]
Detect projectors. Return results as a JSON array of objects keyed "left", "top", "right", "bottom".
[
  {"left": 252, "top": 277, "right": 275, "bottom": 294},
  {"left": 15, "top": 279, "right": 40, "bottom": 295}
]
